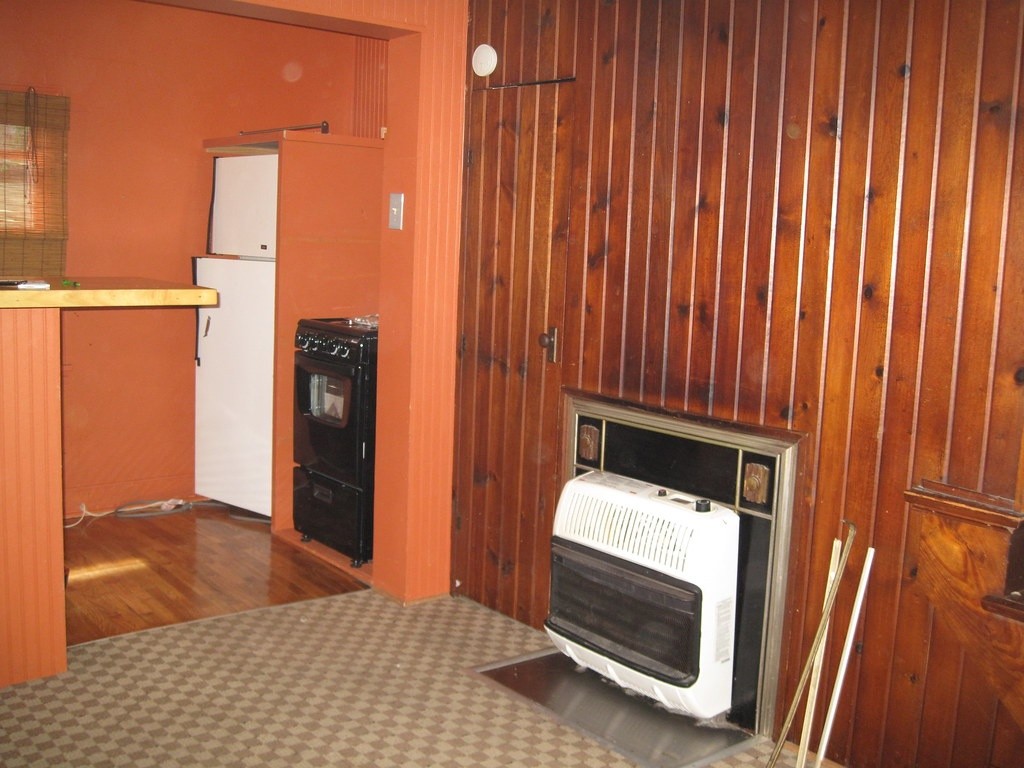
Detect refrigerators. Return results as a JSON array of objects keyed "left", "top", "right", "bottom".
[{"left": 195, "top": 155, "right": 278, "bottom": 522}]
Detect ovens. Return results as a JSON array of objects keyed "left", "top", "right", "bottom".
[{"left": 293, "top": 317, "right": 378, "bottom": 569}]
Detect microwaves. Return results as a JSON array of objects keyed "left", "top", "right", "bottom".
[{"left": 543, "top": 470, "right": 772, "bottom": 730}]
